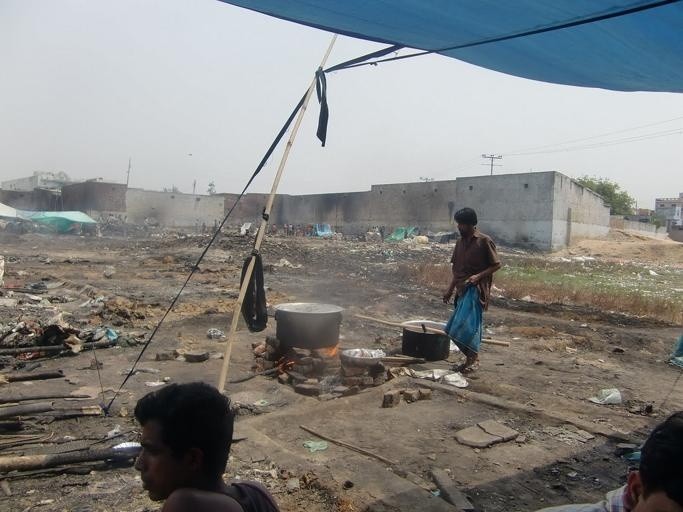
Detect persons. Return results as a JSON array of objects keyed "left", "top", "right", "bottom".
[
  {"left": 194, "top": 219, "right": 221, "bottom": 233},
  {"left": 265, "top": 223, "right": 314, "bottom": 236},
  {"left": 442, "top": 207, "right": 502, "bottom": 375},
  {"left": 534, "top": 410, "right": 683, "bottom": 511},
  {"left": 144, "top": 217, "right": 148, "bottom": 224},
  {"left": 134, "top": 379, "right": 279, "bottom": 512},
  {"left": 97, "top": 213, "right": 128, "bottom": 230},
  {"left": 366, "top": 223, "right": 385, "bottom": 243}
]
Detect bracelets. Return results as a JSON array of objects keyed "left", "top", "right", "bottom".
[{"left": 476, "top": 274, "right": 481, "bottom": 279}]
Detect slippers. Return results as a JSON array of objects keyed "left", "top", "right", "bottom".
[{"left": 453, "top": 363, "right": 480, "bottom": 373}]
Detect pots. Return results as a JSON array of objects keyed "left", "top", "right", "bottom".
[
  {"left": 273, "top": 302, "right": 344, "bottom": 348},
  {"left": 401, "top": 320, "right": 451, "bottom": 362},
  {"left": 341, "top": 348, "right": 424, "bottom": 367}
]
